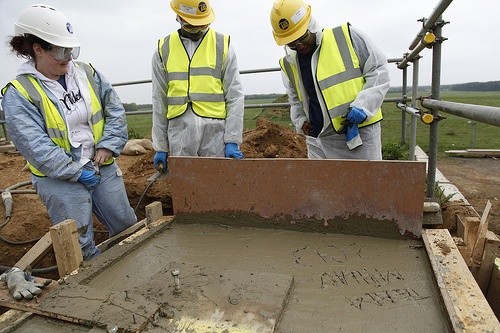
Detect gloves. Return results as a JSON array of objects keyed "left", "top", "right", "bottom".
[
  {"left": 337, "top": 107, "right": 367, "bottom": 151},
  {"left": 4, "top": 266, "right": 51, "bottom": 301},
  {"left": 78, "top": 169, "right": 101, "bottom": 190},
  {"left": 225, "top": 143, "right": 243, "bottom": 159},
  {"left": 153, "top": 151, "right": 168, "bottom": 174}
]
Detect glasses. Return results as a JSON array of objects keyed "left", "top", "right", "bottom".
[
  {"left": 176, "top": 14, "right": 211, "bottom": 34},
  {"left": 48, "top": 45, "right": 81, "bottom": 62},
  {"left": 286, "top": 30, "right": 311, "bottom": 50}
]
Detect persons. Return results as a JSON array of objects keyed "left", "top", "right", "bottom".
[
  {"left": 0, "top": 4, "right": 138, "bottom": 260},
  {"left": 269, "top": 0, "right": 391, "bottom": 160},
  {"left": 151, "top": 0, "right": 245, "bottom": 173}
]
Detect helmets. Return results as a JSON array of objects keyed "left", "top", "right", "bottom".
[
  {"left": 170, "top": 0, "right": 216, "bottom": 26},
  {"left": 12, "top": 4, "right": 81, "bottom": 48},
  {"left": 270, "top": 0, "right": 312, "bottom": 46}
]
